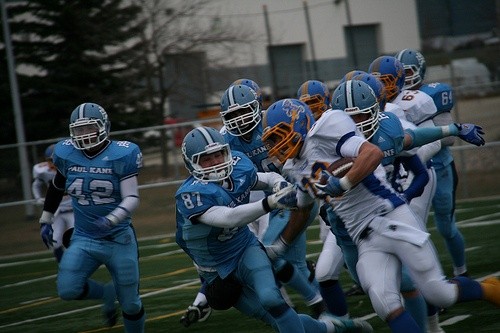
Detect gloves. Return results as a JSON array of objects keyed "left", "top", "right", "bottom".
[
  {"left": 266, "top": 185, "right": 298, "bottom": 211},
  {"left": 96, "top": 218, "right": 112, "bottom": 233},
  {"left": 40, "top": 223, "right": 54, "bottom": 250},
  {"left": 452, "top": 122, "right": 485, "bottom": 147},
  {"left": 315, "top": 171, "right": 354, "bottom": 198},
  {"left": 272, "top": 182, "right": 293, "bottom": 194}
]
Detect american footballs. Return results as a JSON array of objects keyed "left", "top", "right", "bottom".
[{"left": 320, "top": 156, "right": 357, "bottom": 186}]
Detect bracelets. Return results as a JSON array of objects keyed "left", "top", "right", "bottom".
[
  {"left": 39, "top": 211, "right": 53, "bottom": 224},
  {"left": 340, "top": 176, "right": 350, "bottom": 191},
  {"left": 106, "top": 214, "right": 118, "bottom": 226}
]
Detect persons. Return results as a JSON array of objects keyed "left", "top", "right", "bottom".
[
  {"left": 175, "top": 48, "right": 500, "bottom": 333},
  {"left": 38, "top": 104, "right": 145, "bottom": 333},
  {"left": 32, "top": 143, "right": 74, "bottom": 262}
]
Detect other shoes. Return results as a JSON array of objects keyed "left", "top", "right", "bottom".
[{"left": 306, "top": 261, "right": 316, "bottom": 282}]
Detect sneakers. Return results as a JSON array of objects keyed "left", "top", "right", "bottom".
[
  {"left": 179, "top": 306, "right": 201, "bottom": 327},
  {"left": 318, "top": 312, "right": 374, "bottom": 333},
  {"left": 342, "top": 284, "right": 363, "bottom": 294},
  {"left": 101, "top": 280, "right": 117, "bottom": 327}
]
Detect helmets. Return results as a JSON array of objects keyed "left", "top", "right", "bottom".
[
  {"left": 45, "top": 144, "right": 58, "bottom": 169},
  {"left": 231, "top": 79, "right": 262, "bottom": 105},
  {"left": 369, "top": 56, "right": 406, "bottom": 101},
  {"left": 339, "top": 71, "right": 365, "bottom": 85},
  {"left": 221, "top": 85, "right": 262, "bottom": 137},
  {"left": 262, "top": 99, "right": 316, "bottom": 167},
  {"left": 331, "top": 80, "right": 378, "bottom": 142},
  {"left": 351, "top": 73, "right": 387, "bottom": 111},
  {"left": 69, "top": 103, "right": 109, "bottom": 150},
  {"left": 297, "top": 79, "right": 329, "bottom": 119},
  {"left": 181, "top": 127, "right": 234, "bottom": 182},
  {"left": 395, "top": 49, "right": 426, "bottom": 90}
]
